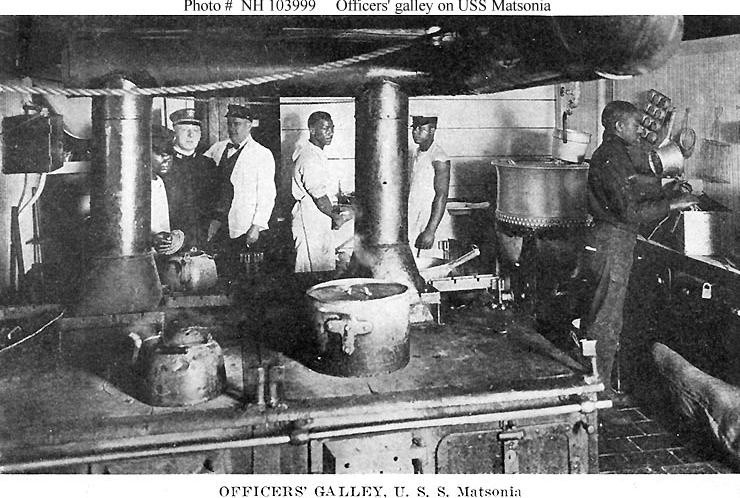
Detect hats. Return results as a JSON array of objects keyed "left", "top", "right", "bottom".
[
  {"left": 224, "top": 104, "right": 252, "bottom": 122},
  {"left": 170, "top": 108, "right": 203, "bottom": 128},
  {"left": 410, "top": 112, "right": 440, "bottom": 127}
]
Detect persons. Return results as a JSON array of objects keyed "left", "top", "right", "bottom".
[
  {"left": 148, "top": 123, "right": 182, "bottom": 263},
  {"left": 581, "top": 101, "right": 701, "bottom": 404},
  {"left": 405, "top": 114, "right": 456, "bottom": 274},
  {"left": 164, "top": 104, "right": 234, "bottom": 259},
  {"left": 289, "top": 109, "right": 344, "bottom": 273},
  {"left": 202, "top": 105, "right": 278, "bottom": 275}
]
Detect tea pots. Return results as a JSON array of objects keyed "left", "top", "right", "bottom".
[
  {"left": 124, "top": 320, "right": 233, "bottom": 409},
  {"left": 159, "top": 249, "right": 220, "bottom": 295}
]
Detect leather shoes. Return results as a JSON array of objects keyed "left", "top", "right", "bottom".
[{"left": 597, "top": 387, "right": 636, "bottom": 426}]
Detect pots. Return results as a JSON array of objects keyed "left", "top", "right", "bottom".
[
  {"left": 646, "top": 135, "right": 685, "bottom": 178},
  {"left": 302, "top": 272, "right": 414, "bottom": 378}
]
